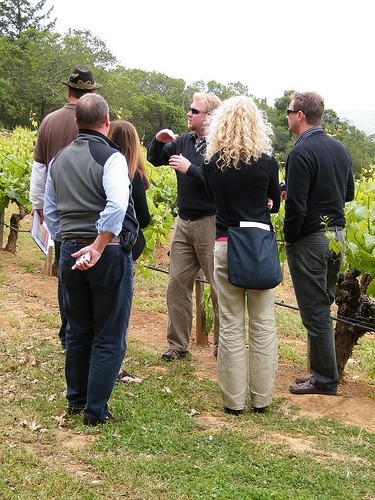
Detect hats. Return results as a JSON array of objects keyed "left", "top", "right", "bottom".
[{"left": 61, "top": 65, "right": 102, "bottom": 89}]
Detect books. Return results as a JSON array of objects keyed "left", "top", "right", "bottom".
[{"left": 30, "top": 209, "right": 51, "bottom": 255}]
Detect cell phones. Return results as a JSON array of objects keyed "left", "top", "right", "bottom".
[{"left": 76, "top": 252, "right": 91, "bottom": 266}]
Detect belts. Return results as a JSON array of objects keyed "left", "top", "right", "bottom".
[
  {"left": 62, "top": 237, "right": 122, "bottom": 244},
  {"left": 318, "top": 226, "right": 344, "bottom": 231}
]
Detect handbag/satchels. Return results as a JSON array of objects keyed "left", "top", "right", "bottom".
[{"left": 227, "top": 227, "right": 282, "bottom": 291}]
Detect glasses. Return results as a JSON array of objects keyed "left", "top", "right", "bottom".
[
  {"left": 287, "top": 110, "right": 298, "bottom": 115},
  {"left": 189, "top": 108, "right": 207, "bottom": 114}
]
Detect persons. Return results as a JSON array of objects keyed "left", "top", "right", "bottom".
[
  {"left": 30, "top": 63, "right": 104, "bottom": 351},
  {"left": 99, "top": 119, "right": 151, "bottom": 384},
  {"left": 275, "top": 91, "right": 356, "bottom": 395},
  {"left": 198, "top": 95, "right": 280, "bottom": 415},
  {"left": 43, "top": 93, "right": 134, "bottom": 428},
  {"left": 145, "top": 90, "right": 223, "bottom": 361}
]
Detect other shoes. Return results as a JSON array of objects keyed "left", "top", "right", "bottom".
[
  {"left": 225, "top": 407, "right": 242, "bottom": 415},
  {"left": 84, "top": 411, "right": 126, "bottom": 426},
  {"left": 289, "top": 379, "right": 338, "bottom": 395},
  {"left": 254, "top": 406, "right": 268, "bottom": 412},
  {"left": 162, "top": 349, "right": 189, "bottom": 362},
  {"left": 295, "top": 374, "right": 314, "bottom": 384},
  {"left": 116, "top": 369, "right": 142, "bottom": 384}
]
